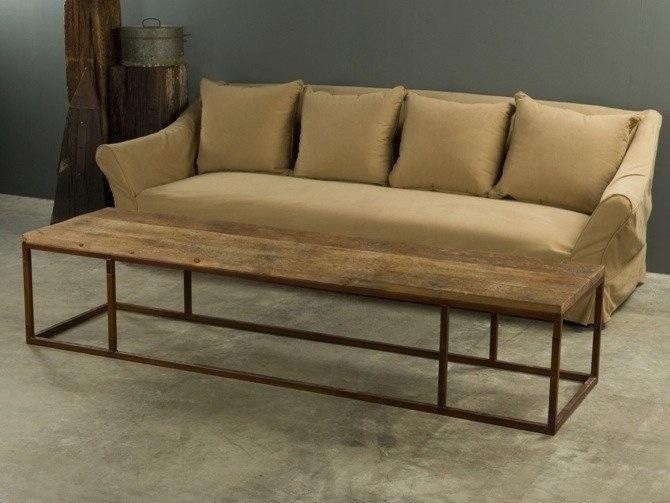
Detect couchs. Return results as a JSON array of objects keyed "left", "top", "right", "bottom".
[{"left": 94, "top": 76, "right": 664, "bottom": 327}]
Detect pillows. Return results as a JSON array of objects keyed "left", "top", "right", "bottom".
[
  {"left": 486, "top": 90, "right": 649, "bottom": 213},
  {"left": 387, "top": 91, "right": 515, "bottom": 196},
  {"left": 296, "top": 84, "right": 407, "bottom": 187},
  {"left": 196, "top": 76, "right": 304, "bottom": 175}
]
